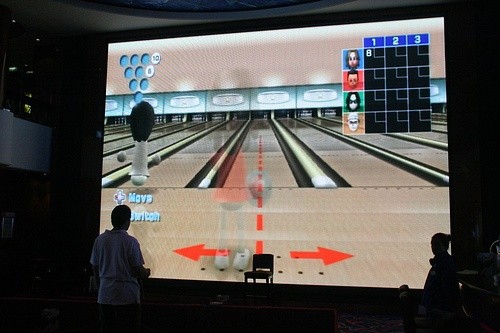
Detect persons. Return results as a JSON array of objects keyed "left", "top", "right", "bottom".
[
  {"left": 87, "top": 205, "right": 150, "bottom": 333},
  {"left": 417, "top": 231, "right": 461, "bottom": 333}
]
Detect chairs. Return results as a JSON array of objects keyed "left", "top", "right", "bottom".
[{"left": 244, "top": 253, "right": 274, "bottom": 283}]
